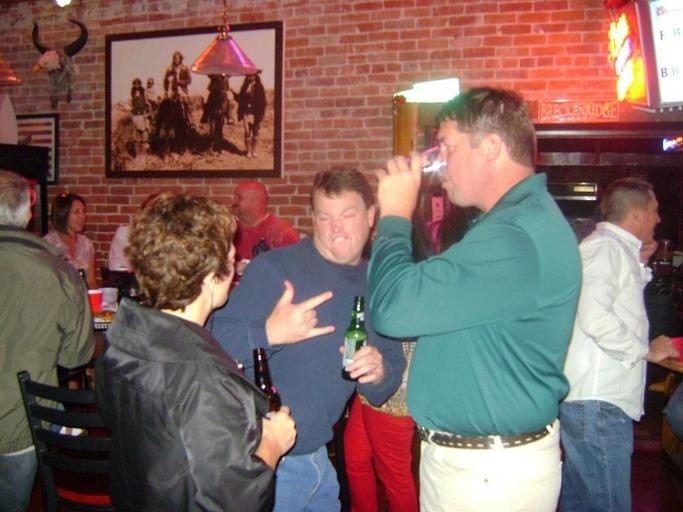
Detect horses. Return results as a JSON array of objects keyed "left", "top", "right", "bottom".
[
  {"left": 207, "top": 74, "right": 229, "bottom": 155},
  {"left": 154, "top": 68, "right": 186, "bottom": 155}
]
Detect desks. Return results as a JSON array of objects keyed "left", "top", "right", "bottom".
[
  {"left": 0, "top": 141, "right": 51, "bottom": 238},
  {"left": 644, "top": 350, "right": 682, "bottom": 468}
]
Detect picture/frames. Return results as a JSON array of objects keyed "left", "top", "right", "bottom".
[
  {"left": 100, "top": 18, "right": 285, "bottom": 180},
  {"left": 14, "top": 110, "right": 60, "bottom": 187}
]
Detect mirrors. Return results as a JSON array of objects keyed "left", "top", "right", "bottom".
[{"left": 390, "top": 76, "right": 461, "bottom": 259}]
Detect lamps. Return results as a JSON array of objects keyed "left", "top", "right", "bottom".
[{"left": 186, "top": 0, "right": 261, "bottom": 78}]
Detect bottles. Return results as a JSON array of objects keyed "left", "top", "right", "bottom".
[
  {"left": 252, "top": 347, "right": 282, "bottom": 419},
  {"left": 339, "top": 295, "right": 369, "bottom": 381}
]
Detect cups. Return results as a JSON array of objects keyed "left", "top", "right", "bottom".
[
  {"left": 74, "top": 267, "right": 118, "bottom": 319},
  {"left": 653, "top": 239, "right": 672, "bottom": 261},
  {"left": 406, "top": 146, "right": 448, "bottom": 189}
]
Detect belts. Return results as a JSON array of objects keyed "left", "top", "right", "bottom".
[{"left": 411, "top": 415, "right": 561, "bottom": 456}]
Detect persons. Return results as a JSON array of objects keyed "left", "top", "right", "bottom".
[
  {"left": 229, "top": 181, "right": 299, "bottom": 292},
  {"left": 365, "top": 86, "right": 583, "bottom": 512},
  {"left": 559, "top": 177, "right": 680, "bottom": 512},
  {"left": 203, "top": 165, "right": 407, "bottom": 512},
  {"left": 131, "top": 50, "right": 265, "bottom": 158},
  {"left": 661, "top": 337, "right": 683, "bottom": 443},
  {"left": 103, "top": 190, "right": 299, "bottom": 512},
  {"left": 1, "top": 169, "right": 95, "bottom": 511},
  {"left": 103, "top": 192, "right": 162, "bottom": 307},
  {"left": 343, "top": 208, "right": 437, "bottom": 511},
  {"left": 40, "top": 193, "right": 96, "bottom": 290}
]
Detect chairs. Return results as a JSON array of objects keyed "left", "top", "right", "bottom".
[
  {"left": 13, "top": 368, "right": 113, "bottom": 511},
  {"left": 97, "top": 266, "right": 148, "bottom": 309}
]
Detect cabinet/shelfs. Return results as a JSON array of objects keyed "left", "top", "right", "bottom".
[{"left": 528, "top": 116, "right": 683, "bottom": 410}]
